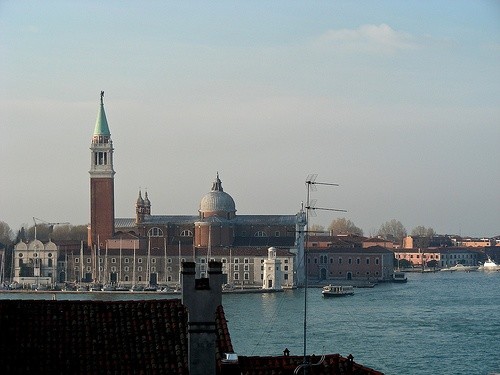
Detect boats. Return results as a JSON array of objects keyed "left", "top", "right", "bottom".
[
  {"left": 479, "top": 256, "right": 500, "bottom": 271},
  {"left": 355, "top": 282, "right": 377, "bottom": 288},
  {"left": 392, "top": 271, "right": 409, "bottom": 282},
  {"left": 322, "top": 284, "right": 355, "bottom": 296},
  {"left": 439, "top": 264, "right": 479, "bottom": 272},
  {"left": 1, "top": 227, "right": 181, "bottom": 293}
]
